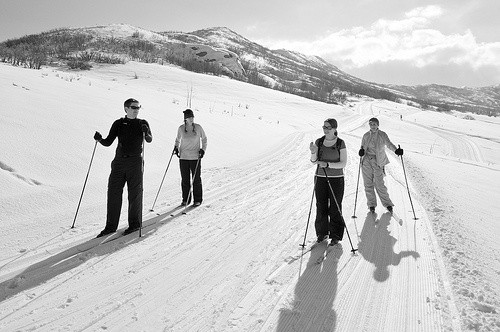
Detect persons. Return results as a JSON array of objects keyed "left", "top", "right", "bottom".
[
  {"left": 359, "top": 118, "right": 403, "bottom": 213},
  {"left": 309, "top": 119, "right": 348, "bottom": 246},
  {"left": 93, "top": 98, "right": 152, "bottom": 238},
  {"left": 173, "top": 109, "right": 208, "bottom": 206}
]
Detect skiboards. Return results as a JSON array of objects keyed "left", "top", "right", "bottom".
[
  {"left": 46, "top": 219, "right": 156, "bottom": 256},
  {"left": 284, "top": 236, "right": 343, "bottom": 265},
  {"left": 168, "top": 190, "right": 225, "bottom": 217},
  {"left": 370, "top": 210, "right": 404, "bottom": 226}
]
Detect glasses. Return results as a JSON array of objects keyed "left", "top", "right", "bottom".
[
  {"left": 323, "top": 126, "right": 332, "bottom": 130},
  {"left": 127, "top": 106, "right": 139, "bottom": 109}
]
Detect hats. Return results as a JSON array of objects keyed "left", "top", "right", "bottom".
[{"left": 184, "top": 109, "right": 194, "bottom": 119}]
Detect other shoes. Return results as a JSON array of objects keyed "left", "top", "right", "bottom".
[
  {"left": 101, "top": 228, "right": 116, "bottom": 234},
  {"left": 182, "top": 201, "right": 186, "bottom": 205},
  {"left": 318, "top": 235, "right": 324, "bottom": 241},
  {"left": 370, "top": 207, "right": 375, "bottom": 212},
  {"left": 331, "top": 238, "right": 338, "bottom": 244},
  {"left": 387, "top": 206, "right": 392, "bottom": 212},
  {"left": 195, "top": 201, "right": 200, "bottom": 206},
  {"left": 125, "top": 227, "right": 139, "bottom": 233}
]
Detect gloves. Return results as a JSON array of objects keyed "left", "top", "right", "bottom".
[
  {"left": 396, "top": 148, "right": 403, "bottom": 155},
  {"left": 199, "top": 150, "right": 204, "bottom": 158},
  {"left": 142, "top": 124, "right": 149, "bottom": 132},
  {"left": 310, "top": 142, "right": 318, "bottom": 154},
  {"left": 316, "top": 161, "right": 327, "bottom": 168},
  {"left": 176, "top": 151, "right": 180, "bottom": 158},
  {"left": 94, "top": 132, "right": 102, "bottom": 140},
  {"left": 359, "top": 149, "right": 364, "bottom": 156}
]
